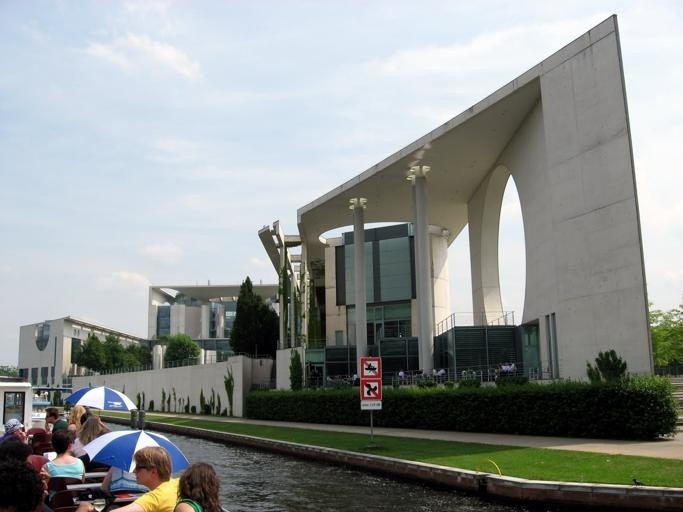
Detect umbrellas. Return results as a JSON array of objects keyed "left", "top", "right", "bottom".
[
  {"left": 82, "top": 428, "right": 191, "bottom": 478},
  {"left": 63, "top": 383, "right": 138, "bottom": 412}
]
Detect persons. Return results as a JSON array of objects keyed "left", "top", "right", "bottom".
[
  {"left": 109, "top": 447, "right": 181, "bottom": 512},
  {"left": 101, "top": 468, "right": 137, "bottom": 493},
  {"left": 171, "top": 461, "right": 224, "bottom": 511},
  {"left": 0, "top": 402, "right": 110, "bottom": 512}
]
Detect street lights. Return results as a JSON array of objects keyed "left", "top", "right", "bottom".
[
  {"left": 348, "top": 196, "right": 370, "bottom": 386},
  {"left": 406, "top": 163, "right": 438, "bottom": 383}
]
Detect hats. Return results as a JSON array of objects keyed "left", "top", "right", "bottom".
[{"left": 5, "top": 419, "right": 25, "bottom": 433}]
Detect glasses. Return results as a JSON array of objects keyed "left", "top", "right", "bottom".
[{"left": 136, "top": 464, "right": 154, "bottom": 471}]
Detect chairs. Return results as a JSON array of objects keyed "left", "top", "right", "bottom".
[{"left": 27, "top": 427, "right": 148, "bottom": 512}]
